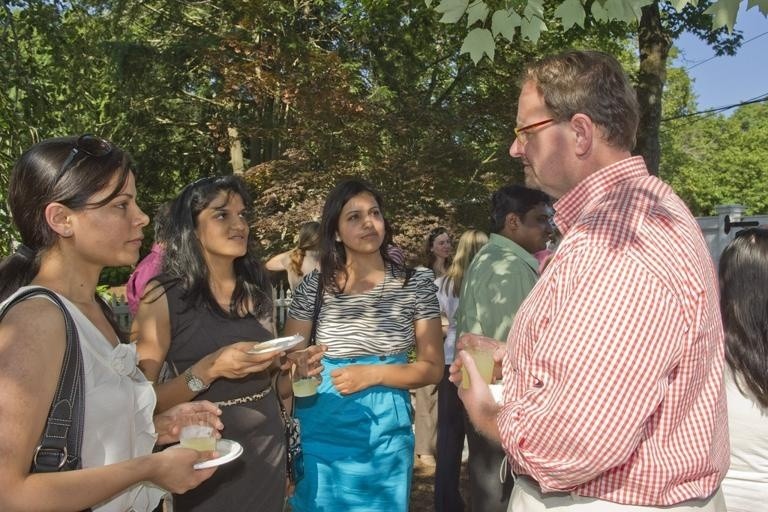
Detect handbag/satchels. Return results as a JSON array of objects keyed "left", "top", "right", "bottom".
[
  {"left": 36, "top": 451, "right": 96, "bottom": 512},
  {"left": 285, "top": 417, "right": 303, "bottom": 486}
]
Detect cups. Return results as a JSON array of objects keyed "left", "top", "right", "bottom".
[
  {"left": 175, "top": 403, "right": 220, "bottom": 452},
  {"left": 288, "top": 351, "right": 318, "bottom": 398},
  {"left": 457, "top": 334, "right": 499, "bottom": 388}
]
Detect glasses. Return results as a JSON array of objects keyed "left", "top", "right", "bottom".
[
  {"left": 513, "top": 120, "right": 558, "bottom": 142},
  {"left": 51, "top": 133, "right": 112, "bottom": 191},
  {"left": 431, "top": 225, "right": 445, "bottom": 235}
]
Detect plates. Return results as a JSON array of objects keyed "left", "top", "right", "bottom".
[
  {"left": 248, "top": 334, "right": 305, "bottom": 355},
  {"left": 160, "top": 438, "right": 243, "bottom": 469}
]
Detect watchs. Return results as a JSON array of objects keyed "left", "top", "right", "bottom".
[{"left": 184, "top": 368, "right": 210, "bottom": 393}]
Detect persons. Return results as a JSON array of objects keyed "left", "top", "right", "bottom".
[
  {"left": 126, "top": 199, "right": 171, "bottom": 315},
  {"left": 713, "top": 222, "right": 768, "bottom": 512},
  {"left": 286, "top": 178, "right": 446, "bottom": 512},
  {"left": 0, "top": 133, "right": 227, "bottom": 512},
  {"left": 449, "top": 46, "right": 736, "bottom": 512},
  {"left": 415, "top": 185, "right": 554, "bottom": 512},
  {"left": 131, "top": 177, "right": 328, "bottom": 512},
  {"left": 265, "top": 222, "right": 322, "bottom": 292}
]
